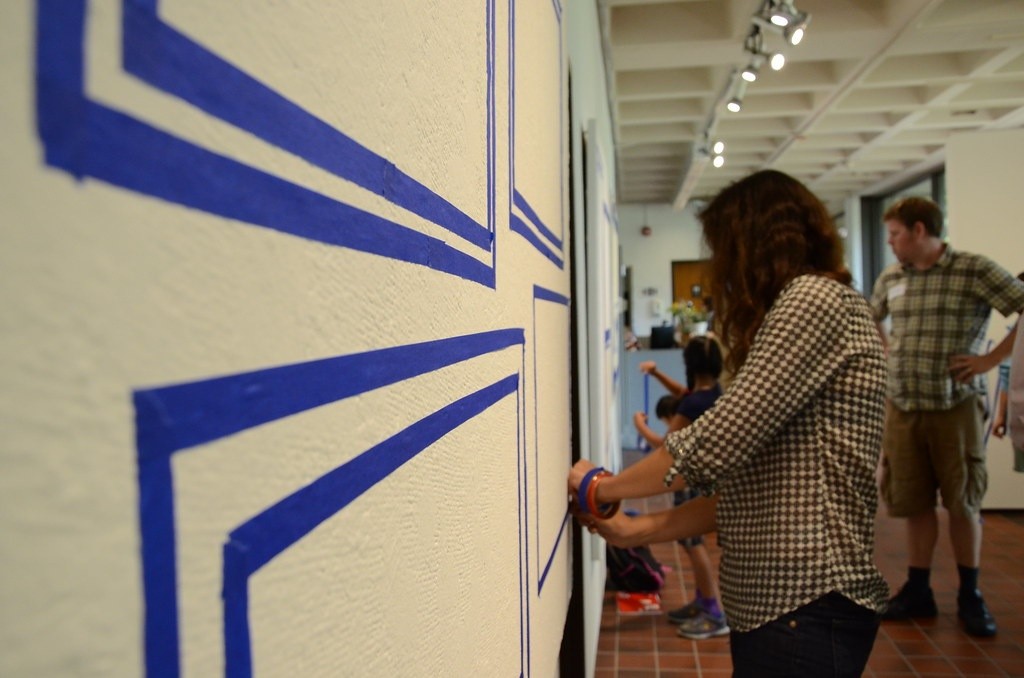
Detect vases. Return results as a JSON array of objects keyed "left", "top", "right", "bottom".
[
  {"left": 690, "top": 321, "right": 709, "bottom": 336},
  {"left": 681, "top": 333, "right": 689, "bottom": 346}
]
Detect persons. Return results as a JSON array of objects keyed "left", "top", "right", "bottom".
[
  {"left": 655, "top": 395, "right": 679, "bottom": 424},
  {"left": 701, "top": 294, "right": 717, "bottom": 331},
  {"left": 869, "top": 195, "right": 1024, "bottom": 637},
  {"left": 633, "top": 336, "right": 730, "bottom": 639},
  {"left": 564, "top": 169, "right": 889, "bottom": 678}
]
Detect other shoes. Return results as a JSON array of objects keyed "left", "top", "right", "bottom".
[
  {"left": 880, "top": 584, "right": 939, "bottom": 620},
  {"left": 677, "top": 609, "right": 731, "bottom": 640},
  {"left": 668, "top": 598, "right": 705, "bottom": 622},
  {"left": 958, "top": 587, "right": 998, "bottom": 638}
]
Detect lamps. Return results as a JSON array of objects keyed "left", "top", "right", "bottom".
[{"left": 697, "top": 0, "right": 812, "bottom": 168}]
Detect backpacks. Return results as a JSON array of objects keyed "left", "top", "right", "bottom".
[{"left": 606, "top": 532, "right": 669, "bottom": 593}]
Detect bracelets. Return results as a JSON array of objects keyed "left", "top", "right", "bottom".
[{"left": 578, "top": 467, "right": 621, "bottom": 521}]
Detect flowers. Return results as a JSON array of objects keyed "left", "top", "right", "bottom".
[{"left": 667, "top": 299, "right": 714, "bottom": 333}]
